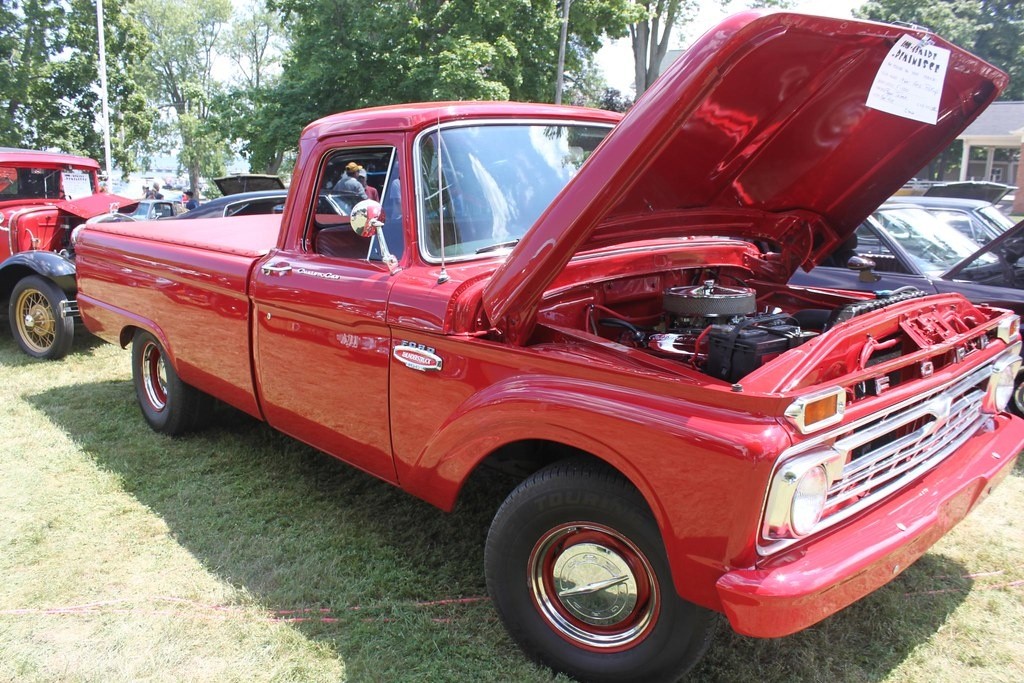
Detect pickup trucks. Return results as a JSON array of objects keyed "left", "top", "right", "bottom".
[{"left": 60, "top": 7, "right": 1023, "bottom": 683}]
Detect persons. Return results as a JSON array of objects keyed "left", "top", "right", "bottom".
[
  {"left": 331, "top": 160, "right": 380, "bottom": 201},
  {"left": 141, "top": 184, "right": 199, "bottom": 211}
]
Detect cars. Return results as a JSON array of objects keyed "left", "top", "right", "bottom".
[
  {"left": 0, "top": 147, "right": 139, "bottom": 362},
  {"left": 156, "top": 188, "right": 364, "bottom": 220},
  {"left": 885, "top": 196, "right": 1023, "bottom": 269},
  {"left": 130, "top": 199, "right": 177, "bottom": 220},
  {"left": 788, "top": 203, "right": 1024, "bottom": 418}
]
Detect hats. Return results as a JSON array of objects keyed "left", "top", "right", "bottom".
[
  {"left": 346, "top": 162, "right": 363, "bottom": 173},
  {"left": 358, "top": 169, "right": 367, "bottom": 178},
  {"left": 184, "top": 191, "right": 194, "bottom": 196},
  {"left": 143, "top": 185, "right": 149, "bottom": 189},
  {"left": 156, "top": 194, "right": 163, "bottom": 199}
]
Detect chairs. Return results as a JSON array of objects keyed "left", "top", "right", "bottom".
[{"left": 315, "top": 225, "right": 371, "bottom": 259}]
